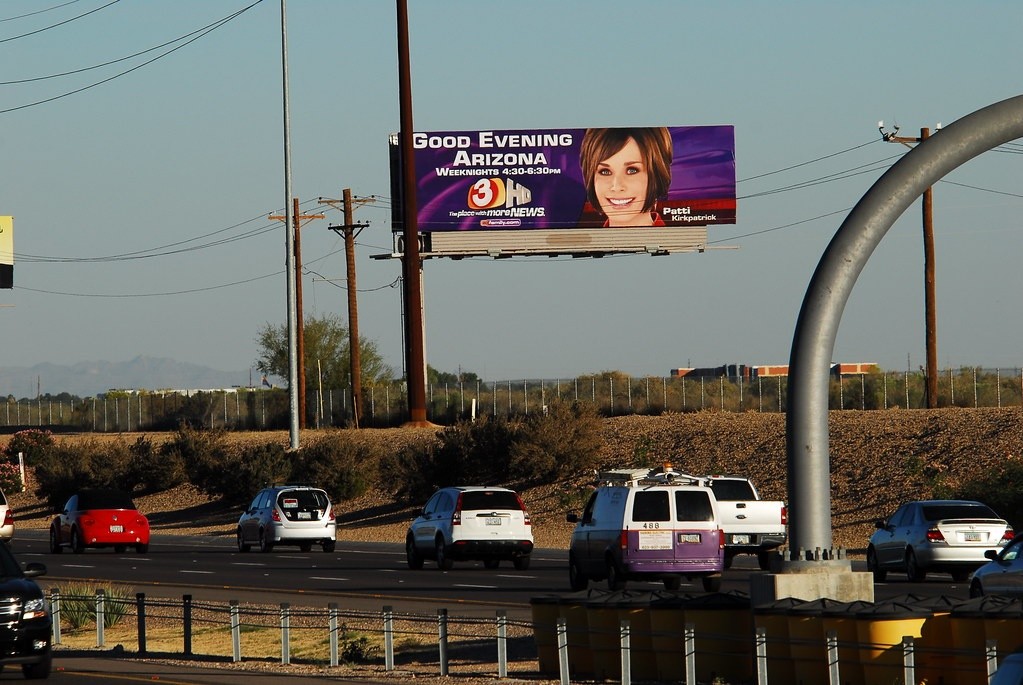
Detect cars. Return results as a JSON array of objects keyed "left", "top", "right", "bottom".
[
  {"left": 50, "top": 490, "right": 149, "bottom": 552},
  {"left": 0, "top": 545, "right": 52, "bottom": 679},
  {"left": 866, "top": 501, "right": 1015, "bottom": 584},
  {"left": 0, "top": 486, "right": 14, "bottom": 544},
  {"left": 969, "top": 532, "right": 1023, "bottom": 599},
  {"left": 237, "top": 483, "right": 336, "bottom": 554}
]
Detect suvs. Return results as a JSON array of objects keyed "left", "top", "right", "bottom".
[{"left": 406, "top": 486, "right": 534, "bottom": 571}]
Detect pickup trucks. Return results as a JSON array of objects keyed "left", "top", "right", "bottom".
[{"left": 705, "top": 475, "right": 787, "bottom": 568}]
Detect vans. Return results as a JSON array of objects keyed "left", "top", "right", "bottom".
[{"left": 567, "top": 464, "right": 724, "bottom": 594}]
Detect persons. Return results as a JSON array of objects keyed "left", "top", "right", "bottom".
[{"left": 579, "top": 127, "right": 673, "bottom": 227}]
